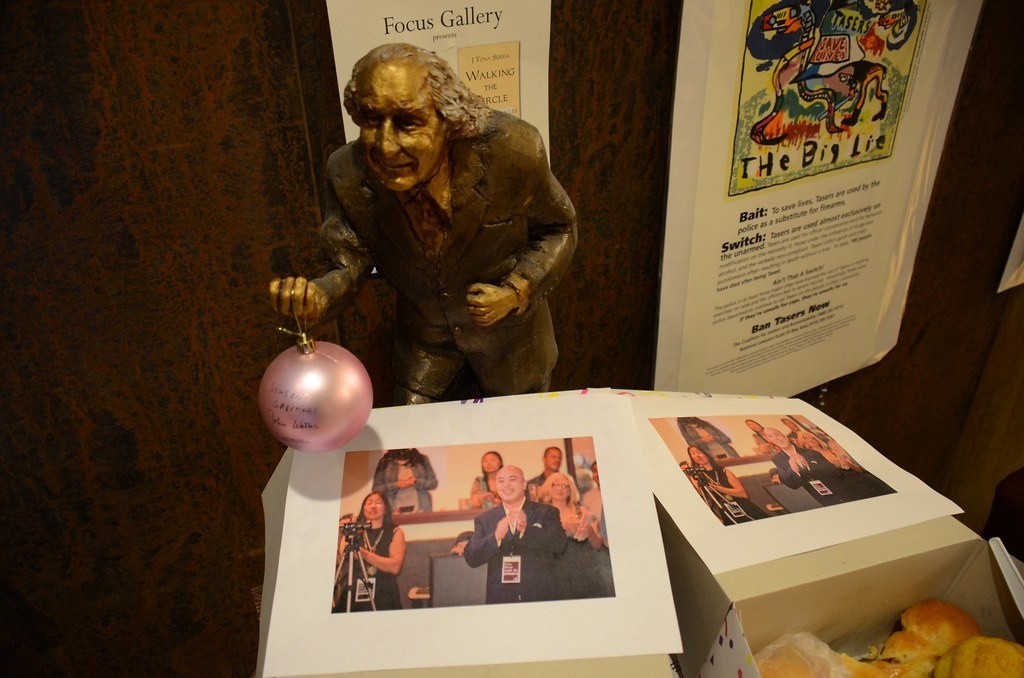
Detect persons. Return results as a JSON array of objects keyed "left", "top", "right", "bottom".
[
  {"left": 266, "top": 45, "right": 580, "bottom": 408},
  {"left": 332, "top": 447, "right": 616, "bottom": 615},
  {"left": 680, "top": 418, "right": 897, "bottom": 526}
]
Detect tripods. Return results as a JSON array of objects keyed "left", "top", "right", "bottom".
[
  {"left": 334, "top": 538, "right": 376, "bottom": 613},
  {"left": 694, "top": 474, "right": 756, "bottom": 526}
]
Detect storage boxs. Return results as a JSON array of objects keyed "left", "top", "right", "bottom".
[{"left": 257, "top": 389, "right": 1024, "bottom": 678}]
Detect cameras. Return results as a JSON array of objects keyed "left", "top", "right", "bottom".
[
  {"left": 343, "top": 523, "right": 372, "bottom": 538},
  {"left": 687, "top": 465, "right": 706, "bottom": 477}
]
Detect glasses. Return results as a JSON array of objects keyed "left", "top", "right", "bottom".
[
  {"left": 552, "top": 483, "right": 570, "bottom": 488},
  {"left": 803, "top": 435, "right": 813, "bottom": 438}
]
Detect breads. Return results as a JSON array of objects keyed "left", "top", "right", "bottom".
[{"left": 750, "top": 596, "right": 1024, "bottom": 678}]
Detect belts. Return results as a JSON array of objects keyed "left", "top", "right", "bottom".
[
  {"left": 399, "top": 506, "right": 414, "bottom": 512},
  {"left": 717, "top": 454, "right": 727, "bottom": 459}
]
